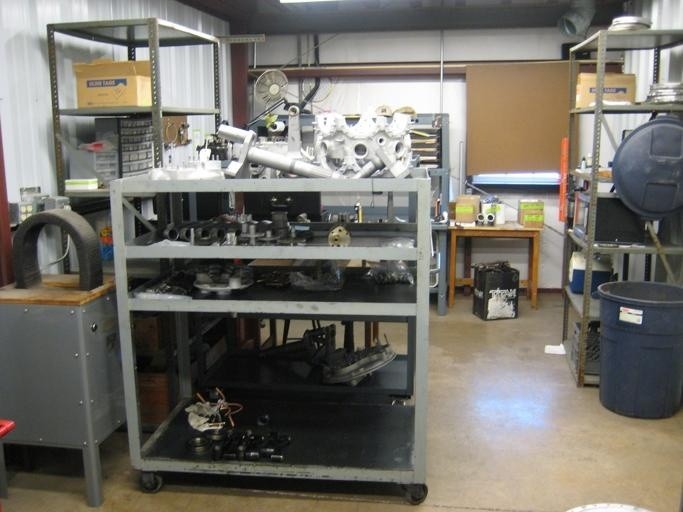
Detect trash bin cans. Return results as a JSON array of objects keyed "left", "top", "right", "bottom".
[{"left": 473, "top": 265, "right": 519, "bottom": 320}]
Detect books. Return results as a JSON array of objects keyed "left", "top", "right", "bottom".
[
  {"left": 647, "top": 96, "right": 683, "bottom": 104},
  {"left": 649, "top": 83, "right": 683, "bottom": 90},
  {"left": 409, "top": 124, "right": 441, "bottom": 171},
  {"left": 648, "top": 89, "right": 683, "bottom": 97}
]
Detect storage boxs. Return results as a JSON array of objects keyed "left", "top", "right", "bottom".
[{"left": 576, "top": 72, "right": 635, "bottom": 107}]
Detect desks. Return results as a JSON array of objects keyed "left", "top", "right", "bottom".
[{"left": 448, "top": 220, "right": 542, "bottom": 310}]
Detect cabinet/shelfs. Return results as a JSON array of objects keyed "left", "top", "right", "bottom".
[
  {"left": 47, "top": 16, "right": 233, "bottom": 388},
  {"left": 562, "top": 30, "right": 683, "bottom": 387}
]
